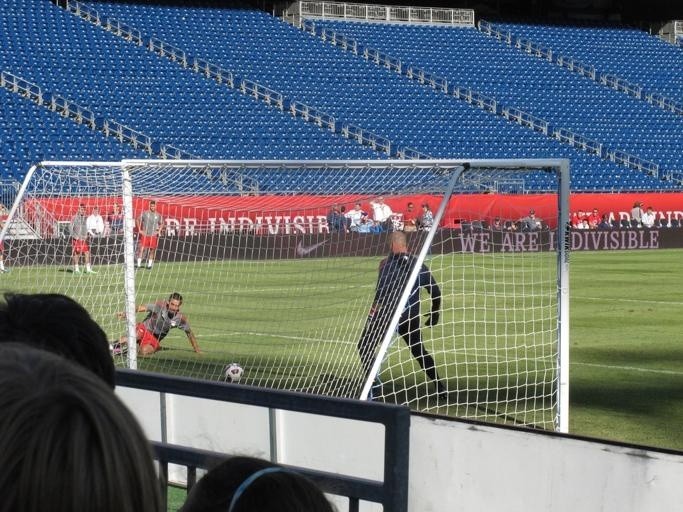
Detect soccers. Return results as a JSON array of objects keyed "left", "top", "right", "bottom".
[{"left": 223, "top": 362, "right": 244, "bottom": 382}]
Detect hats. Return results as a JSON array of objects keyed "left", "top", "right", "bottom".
[
  {"left": 495, "top": 216, "right": 500, "bottom": 221},
  {"left": 529, "top": 209, "right": 535, "bottom": 214}
]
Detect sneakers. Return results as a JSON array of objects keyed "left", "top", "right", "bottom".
[
  {"left": 88, "top": 271, "right": 97, "bottom": 275},
  {"left": 136, "top": 265, "right": 141, "bottom": 267},
  {"left": 147, "top": 264, "right": 151, "bottom": 268},
  {"left": 434, "top": 387, "right": 449, "bottom": 402},
  {"left": 74, "top": 271, "right": 83, "bottom": 274},
  {"left": 109, "top": 340, "right": 121, "bottom": 350},
  {"left": 1, "top": 269, "right": 8, "bottom": 273}
]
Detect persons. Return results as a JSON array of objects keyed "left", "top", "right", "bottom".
[
  {"left": 334, "top": 206, "right": 350, "bottom": 234},
  {"left": 0, "top": 341, "right": 163, "bottom": 511},
  {"left": 369, "top": 196, "right": 392, "bottom": 225},
  {"left": 401, "top": 203, "right": 418, "bottom": 232},
  {"left": 356, "top": 231, "right": 450, "bottom": 404},
  {"left": 0, "top": 206, "right": 14, "bottom": 274},
  {"left": 642, "top": 208, "right": 656, "bottom": 228},
  {"left": 110, "top": 202, "right": 124, "bottom": 235},
  {"left": 136, "top": 200, "right": 165, "bottom": 269},
  {"left": 346, "top": 202, "right": 365, "bottom": 226},
  {"left": 175, "top": 456, "right": 333, "bottom": 512},
  {"left": 520, "top": 209, "right": 543, "bottom": 231},
  {"left": 493, "top": 216, "right": 502, "bottom": 231},
  {"left": 354, "top": 211, "right": 374, "bottom": 232},
  {"left": 68, "top": 204, "right": 99, "bottom": 275},
  {"left": 567, "top": 208, "right": 611, "bottom": 231},
  {"left": 631, "top": 201, "right": 644, "bottom": 219},
  {"left": 86, "top": 206, "right": 104, "bottom": 236},
  {"left": 326, "top": 202, "right": 340, "bottom": 229},
  {"left": 0, "top": 293, "right": 117, "bottom": 389},
  {"left": 108, "top": 291, "right": 203, "bottom": 358},
  {"left": 419, "top": 203, "right": 435, "bottom": 226}
]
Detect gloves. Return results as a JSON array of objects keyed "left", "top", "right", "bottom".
[{"left": 424, "top": 310, "right": 440, "bottom": 328}]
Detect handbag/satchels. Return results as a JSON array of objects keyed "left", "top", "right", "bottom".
[{"left": 639, "top": 208, "right": 644, "bottom": 227}]
